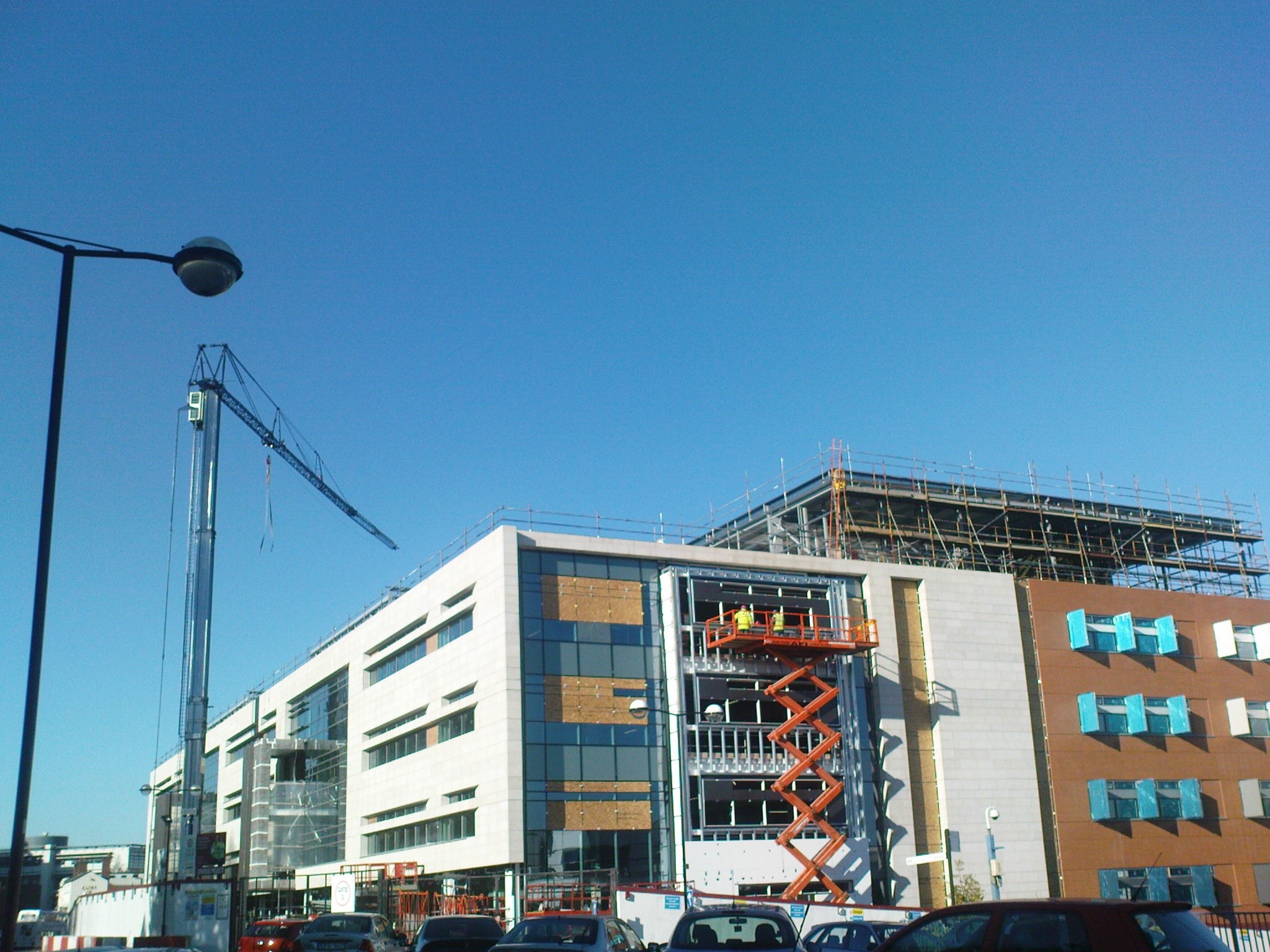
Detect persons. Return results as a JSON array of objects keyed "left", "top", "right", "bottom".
[
  {"left": 734, "top": 605, "right": 753, "bottom": 633},
  {"left": 771, "top": 610, "right": 784, "bottom": 637}
]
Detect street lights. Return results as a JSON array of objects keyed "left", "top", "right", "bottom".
[
  {"left": 139, "top": 783, "right": 201, "bottom": 936},
  {"left": 0, "top": 220, "right": 246, "bottom": 952},
  {"left": 629, "top": 699, "right": 724, "bottom": 912},
  {"left": 985, "top": 806, "right": 1002, "bottom": 901}
]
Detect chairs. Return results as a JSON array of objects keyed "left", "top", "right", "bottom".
[
  {"left": 692, "top": 924, "right": 720, "bottom": 945},
  {"left": 752, "top": 922, "right": 780, "bottom": 944},
  {"left": 573, "top": 934, "right": 590, "bottom": 944},
  {"left": 826, "top": 935, "right": 840, "bottom": 948},
  {"left": 523, "top": 925, "right": 542, "bottom": 941}
]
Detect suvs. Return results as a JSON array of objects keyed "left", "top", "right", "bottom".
[{"left": 289, "top": 912, "right": 408, "bottom": 952}]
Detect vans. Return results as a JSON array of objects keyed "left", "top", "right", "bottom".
[{"left": 12, "top": 909, "right": 72, "bottom": 952}]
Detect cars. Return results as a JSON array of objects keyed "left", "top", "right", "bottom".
[
  {"left": 867, "top": 896, "right": 1234, "bottom": 952},
  {"left": 802, "top": 920, "right": 947, "bottom": 952},
  {"left": 655, "top": 903, "right": 808, "bottom": 952},
  {"left": 399, "top": 914, "right": 504, "bottom": 952},
  {"left": 488, "top": 913, "right": 659, "bottom": 952},
  {"left": 237, "top": 919, "right": 315, "bottom": 952}
]
